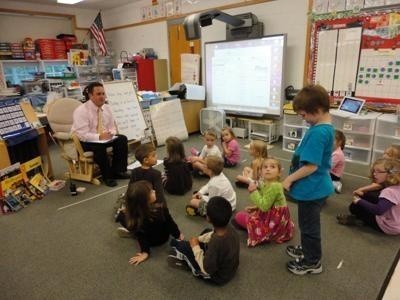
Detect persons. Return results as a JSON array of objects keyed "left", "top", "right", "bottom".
[
  {"left": 113, "top": 126, "right": 296, "bottom": 287},
  {"left": 281, "top": 84, "right": 334, "bottom": 276},
  {"left": 70, "top": 81, "right": 131, "bottom": 187},
  {"left": 356, "top": 144, "right": 400, "bottom": 192},
  {"left": 329, "top": 130, "right": 346, "bottom": 193},
  {"left": 336, "top": 159, "right": 400, "bottom": 236}
]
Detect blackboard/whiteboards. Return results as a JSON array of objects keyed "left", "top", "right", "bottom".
[{"left": 102, "top": 81, "right": 149, "bottom": 145}]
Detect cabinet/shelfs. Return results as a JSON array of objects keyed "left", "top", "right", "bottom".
[
  {"left": 226, "top": 117, "right": 282, "bottom": 145},
  {"left": 330, "top": 104, "right": 377, "bottom": 179},
  {"left": 1, "top": 62, "right": 69, "bottom": 90},
  {"left": 284, "top": 106, "right": 307, "bottom": 153},
  {"left": 373, "top": 113, "right": 399, "bottom": 178},
  {"left": 113, "top": 68, "right": 138, "bottom": 90}
]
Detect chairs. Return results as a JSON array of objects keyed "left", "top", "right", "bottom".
[{"left": 46, "top": 98, "right": 113, "bottom": 185}]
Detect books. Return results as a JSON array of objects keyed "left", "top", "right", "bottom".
[{"left": 0, "top": 155, "right": 65, "bottom": 214}]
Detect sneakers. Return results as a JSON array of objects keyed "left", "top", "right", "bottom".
[
  {"left": 355, "top": 219, "right": 364, "bottom": 227},
  {"left": 287, "top": 244, "right": 305, "bottom": 259},
  {"left": 336, "top": 212, "right": 358, "bottom": 226},
  {"left": 193, "top": 170, "right": 207, "bottom": 178},
  {"left": 185, "top": 205, "right": 201, "bottom": 217},
  {"left": 231, "top": 219, "right": 247, "bottom": 231},
  {"left": 286, "top": 257, "right": 323, "bottom": 276},
  {"left": 235, "top": 182, "right": 250, "bottom": 188},
  {"left": 116, "top": 227, "right": 134, "bottom": 238},
  {"left": 190, "top": 148, "right": 199, "bottom": 156},
  {"left": 332, "top": 180, "right": 343, "bottom": 195},
  {"left": 186, "top": 156, "right": 194, "bottom": 161},
  {"left": 166, "top": 254, "right": 191, "bottom": 271}
]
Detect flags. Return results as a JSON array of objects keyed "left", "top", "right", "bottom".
[{"left": 89, "top": 12, "right": 107, "bottom": 58}]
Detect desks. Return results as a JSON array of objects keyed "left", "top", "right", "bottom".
[{"left": 160, "top": 92, "right": 205, "bottom": 135}]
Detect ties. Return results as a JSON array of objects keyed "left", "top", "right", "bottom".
[{"left": 98, "top": 107, "right": 103, "bottom": 135}]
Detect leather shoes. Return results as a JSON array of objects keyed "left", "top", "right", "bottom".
[
  {"left": 113, "top": 172, "right": 131, "bottom": 180},
  {"left": 101, "top": 175, "right": 117, "bottom": 187}
]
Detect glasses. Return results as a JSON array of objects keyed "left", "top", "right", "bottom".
[{"left": 370, "top": 168, "right": 389, "bottom": 174}]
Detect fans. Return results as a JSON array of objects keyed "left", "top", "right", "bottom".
[{"left": 200, "top": 107, "right": 227, "bottom": 141}]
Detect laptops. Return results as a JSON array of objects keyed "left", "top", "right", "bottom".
[{"left": 331, "top": 96, "right": 366, "bottom": 119}]
